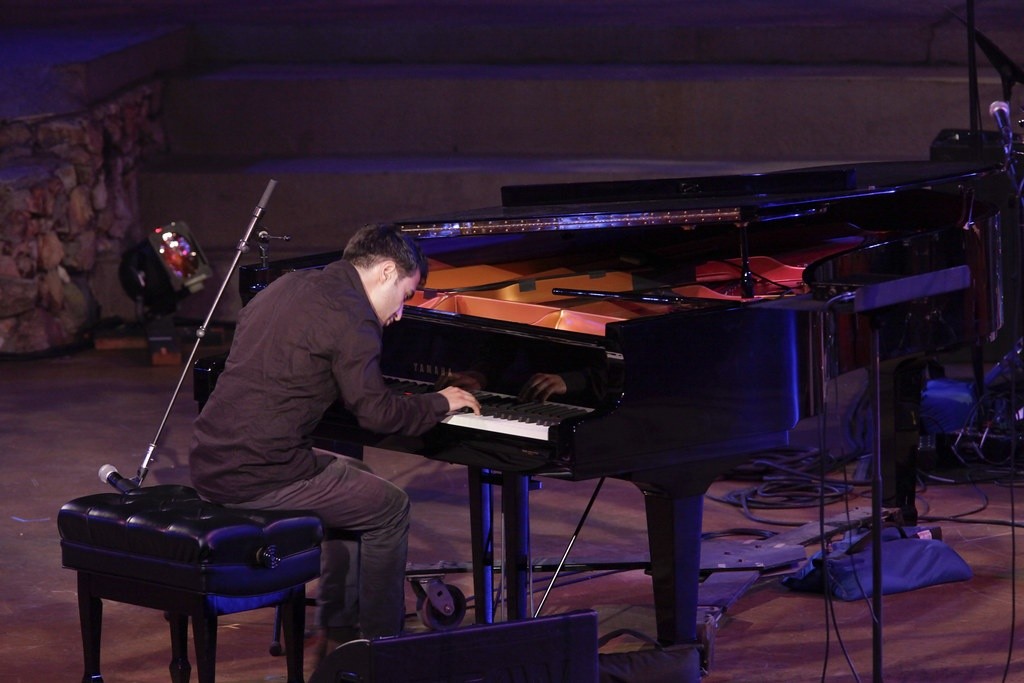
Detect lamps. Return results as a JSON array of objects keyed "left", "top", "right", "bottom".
[{"left": 118, "top": 219, "right": 215, "bottom": 367}]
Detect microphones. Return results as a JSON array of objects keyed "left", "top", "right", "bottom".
[
  {"left": 989, "top": 100, "right": 1013, "bottom": 144},
  {"left": 982, "top": 337, "right": 1024, "bottom": 384},
  {"left": 97, "top": 464, "right": 146, "bottom": 503}
]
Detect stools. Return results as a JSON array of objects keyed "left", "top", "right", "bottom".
[{"left": 54, "top": 485, "right": 326, "bottom": 683}]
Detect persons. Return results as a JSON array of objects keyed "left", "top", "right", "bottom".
[
  {"left": 433, "top": 372, "right": 568, "bottom": 403},
  {"left": 184, "top": 223, "right": 481, "bottom": 656}
]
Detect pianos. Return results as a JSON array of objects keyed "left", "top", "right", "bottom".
[{"left": 188, "top": 152, "right": 1012, "bottom": 653}]
